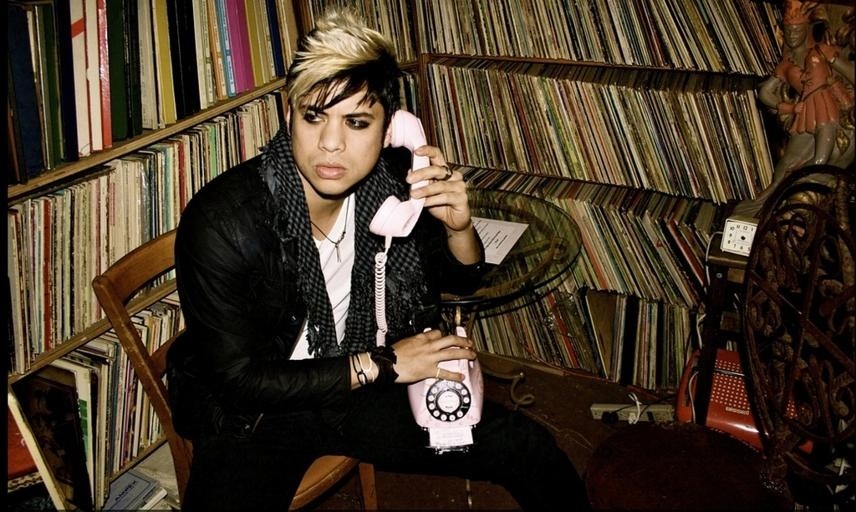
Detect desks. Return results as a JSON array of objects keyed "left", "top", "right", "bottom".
[{"left": 440, "top": 187, "right": 582, "bottom": 409}]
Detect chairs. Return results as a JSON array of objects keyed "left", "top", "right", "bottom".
[{"left": 91, "top": 229, "right": 368, "bottom": 512}]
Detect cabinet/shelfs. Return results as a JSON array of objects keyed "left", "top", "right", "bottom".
[{"left": 0, "top": 0, "right": 788, "bottom": 511}]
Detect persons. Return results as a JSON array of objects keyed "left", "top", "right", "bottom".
[
  {"left": 171, "top": 8, "right": 586, "bottom": 512},
  {"left": 761, "top": 0, "right": 856, "bottom": 170}
]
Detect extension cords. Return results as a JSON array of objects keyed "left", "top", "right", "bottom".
[{"left": 590, "top": 403, "right": 676, "bottom": 423}]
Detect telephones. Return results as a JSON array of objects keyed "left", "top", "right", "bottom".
[{"left": 369, "top": 109, "right": 430, "bottom": 238}]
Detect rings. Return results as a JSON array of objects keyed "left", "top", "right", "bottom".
[{"left": 436, "top": 369, "right": 440, "bottom": 378}]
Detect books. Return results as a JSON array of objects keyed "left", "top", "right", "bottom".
[
  {"left": 3, "top": 293, "right": 188, "bottom": 511},
  {"left": 2, "top": 1, "right": 417, "bottom": 186},
  {"left": 423, "top": 59, "right": 776, "bottom": 208},
  {"left": 5, "top": 90, "right": 289, "bottom": 375},
  {"left": 441, "top": 162, "right": 727, "bottom": 393},
  {"left": 417, "top": 0, "right": 789, "bottom": 77}
]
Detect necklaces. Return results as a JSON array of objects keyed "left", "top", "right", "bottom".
[{"left": 310, "top": 192, "right": 349, "bottom": 261}]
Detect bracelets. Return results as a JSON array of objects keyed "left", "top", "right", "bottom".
[{"left": 352, "top": 345, "right": 399, "bottom": 387}]
